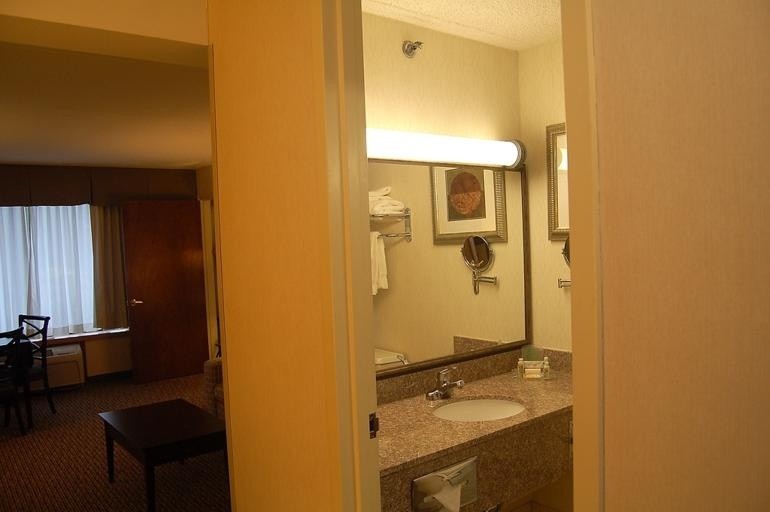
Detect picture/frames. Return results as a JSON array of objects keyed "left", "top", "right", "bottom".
[
  {"left": 429, "top": 167, "right": 508, "bottom": 244},
  {"left": 547, "top": 123, "right": 569, "bottom": 241}
]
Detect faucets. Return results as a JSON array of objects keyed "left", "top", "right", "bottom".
[{"left": 437, "top": 367, "right": 464, "bottom": 391}]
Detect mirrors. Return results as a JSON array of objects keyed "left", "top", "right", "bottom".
[
  {"left": 367, "top": 162, "right": 533, "bottom": 381},
  {"left": 461, "top": 235, "right": 497, "bottom": 294},
  {"left": 557, "top": 236, "right": 571, "bottom": 288}
]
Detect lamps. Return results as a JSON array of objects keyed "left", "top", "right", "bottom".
[{"left": 366, "top": 129, "right": 528, "bottom": 168}]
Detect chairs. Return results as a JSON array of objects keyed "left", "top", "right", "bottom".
[
  {"left": 18, "top": 314, "right": 57, "bottom": 429},
  {"left": 200, "top": 356, "right": 225, "bottom": 421}
]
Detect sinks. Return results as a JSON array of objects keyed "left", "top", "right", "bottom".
[{"left": 432, "top": 395, "right": 526, "bottom": 422}]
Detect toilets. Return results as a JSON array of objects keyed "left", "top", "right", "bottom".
[{"left": 375, "top": 347, "right": 404, "bottom": 373}]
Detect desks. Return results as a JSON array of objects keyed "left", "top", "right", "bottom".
[{"left": 98, "top": 397, "right": 225, "bottom": 512}]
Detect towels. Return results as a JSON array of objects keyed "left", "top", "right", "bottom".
[
  {"left": 369, "top": 187, "right": 404, "bottom": 215},
  {"left": 370, "top": 231, "right": 389, "bottom": 297}
]
